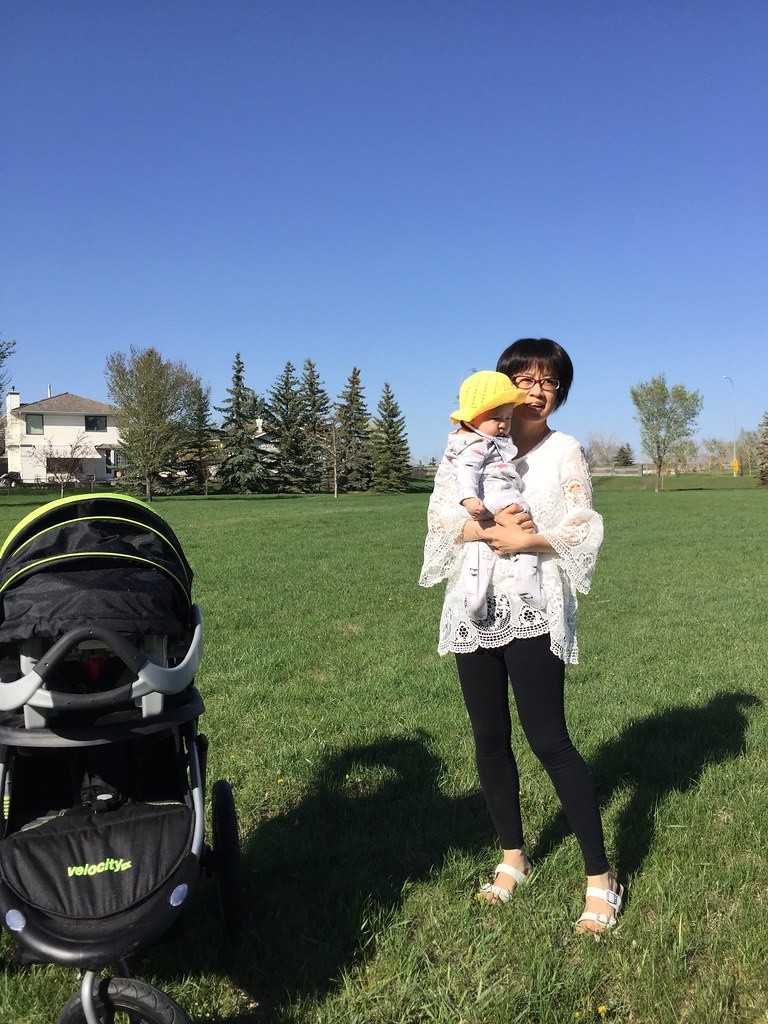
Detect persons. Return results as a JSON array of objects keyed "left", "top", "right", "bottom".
[{"left": 418, "top": 338, "right": 624, "bottom": 938}]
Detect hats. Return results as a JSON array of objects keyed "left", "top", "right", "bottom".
[{"left": 450, "top": 370, "right": 527, "bottom": 427}]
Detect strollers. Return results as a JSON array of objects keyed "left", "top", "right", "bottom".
[{"left": 1, "top": 491, "right": 249, "bottom": 1024}]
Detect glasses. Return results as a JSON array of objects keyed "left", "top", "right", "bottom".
[{"left": 511, "top": 374, "right": 562, "bottom": 391}]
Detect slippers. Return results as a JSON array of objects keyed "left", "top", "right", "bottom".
[
  {"left": 572, "top": 881, "right": 624, "bottom": 941},
  {"left": 477, "top": 861, "right": 538, "bottom": 906}
]
